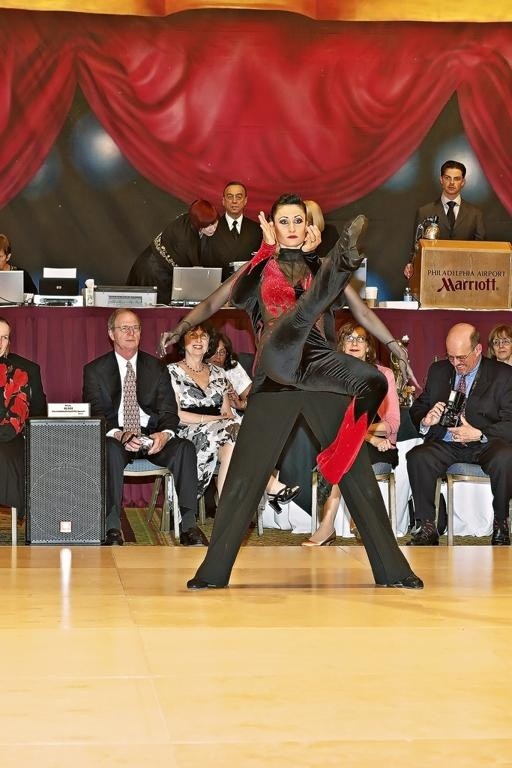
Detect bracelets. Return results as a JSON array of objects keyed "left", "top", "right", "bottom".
[{"left": 200, "top": 415, "right": 204, "bottom": 423}]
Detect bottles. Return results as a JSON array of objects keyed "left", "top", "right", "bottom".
[{"left": 403, "top": 288, "right": 413, "bottom": 301}]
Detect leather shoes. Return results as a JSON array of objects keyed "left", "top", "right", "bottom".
[
  {"left": 179, "top": 527, "right": 209, "bottom": 546},
  {"left": 106, "top": 529, "right": 124, "bottom": 546},
  {"left": 491, "top": 520, "right": 510, "bottom": 546},
  {"left": 374, "top": 573, "right": 424, "bottom": 590},
  {"left": 187, "top": 577, "right": 223, "bottom": 588},
  {"left": 406, "top": 532, "right": 439, "bottom": 546}
]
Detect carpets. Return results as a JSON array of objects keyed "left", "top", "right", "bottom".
[{"left": 4, "top": 510, "right": 511, "bottom": 547}]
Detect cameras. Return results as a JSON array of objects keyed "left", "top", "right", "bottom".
[
  {"left": 137, "top": 435, "right": 154, "bottom": 456},
  {"left": 438, "top": 390, "right": 465, "bottom": 427}
]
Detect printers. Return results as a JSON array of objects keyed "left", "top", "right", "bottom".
[{"left": 34, "top": 279, "right": 84, "bottom": 306}]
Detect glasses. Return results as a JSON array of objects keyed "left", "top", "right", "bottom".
[
  {"left": 214, "top": 349, "right": 226, "bottom": 356},
  {"left": 493, "top": 338, "right": 512, "bottom": 346},
  {"left": 112, "top": 325, "right": 141, "bottom": 334},
  {"left": 446, "top": 348, "right": 474, "bottom": 361},
  {"left": 344, "top": 335, "right": 366, "bottom": 344}
]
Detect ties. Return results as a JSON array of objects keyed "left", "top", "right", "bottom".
[
  {"left": 447, "top": 201, "right": 456, "bottom": 230},
  {"left": 455, "top": 375, "right": 468, "bottom": 448},
  {"left": 231, "top": 221, "right": 238, "bottom": 240},
  {"left": 123, "top": 361, "right": 141, "bottom": 437}
]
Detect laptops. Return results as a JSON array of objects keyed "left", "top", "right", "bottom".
[
  {"left": 170, "top": 267, "right": 222, "bottom": 306},
  {"left": 0, "top": 270, "right": 24, "bottom": 304}
]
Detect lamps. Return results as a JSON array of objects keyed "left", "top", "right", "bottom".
[{"left": 414, "top": 214, "right": 442, "bottom": 245}]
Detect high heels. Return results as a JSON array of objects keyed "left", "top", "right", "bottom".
[
  {"left": 301, "top": 528, "right": 336, "bottom": 547},
  {"left": 339, "top": 214, "right": 365, "bottom": 270},
  {"left": 267, "top": 485, "right": 303, "bottom": 514},
  {"left": 317, "top": 468, "right": 332, "bottom": 508}
]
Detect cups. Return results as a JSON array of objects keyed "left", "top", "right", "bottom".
[{"left": 364, "top": 287, "right": 378, "bottom": 308}]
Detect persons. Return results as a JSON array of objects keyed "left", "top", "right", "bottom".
[
  {"left": 80, "top": 308, "right": 205, "bottom": 547},
  {"left": 405, "top": 323, "right": 512, "bottom": 546},
  {"left": 166, "top": 320, "right": 303, "bottom": 514},
  {"left": 180, "top": 211, "right": 426, "bottom": 592},
  {"left": 126, "top": 181, "right": 340, "bottom": 305},
  {"left": 300, "top": 321, "right": 400, "bottom": 546},
  {"left": 3, "top": 340, "right": 48, "bottom": 420},
  {"left": 1, "top": 233, "right": 40, "bottom": 294},
  {"left": 401, "top": 161, "right": 486, "bottom": 281},
  {"left": 0, "top": 316, "right": 31, "bottom": 521}
]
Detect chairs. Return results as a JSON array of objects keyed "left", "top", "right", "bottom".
[
  {"left": 311, "top": 458, "right": 489, "bottom": 547},
  {"left": 125, "top": 457, "right": 170, "bottom": 537}
]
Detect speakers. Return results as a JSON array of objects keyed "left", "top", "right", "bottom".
[{"left": 26, "top": 416, "right": 106, "bottom": 546}]
